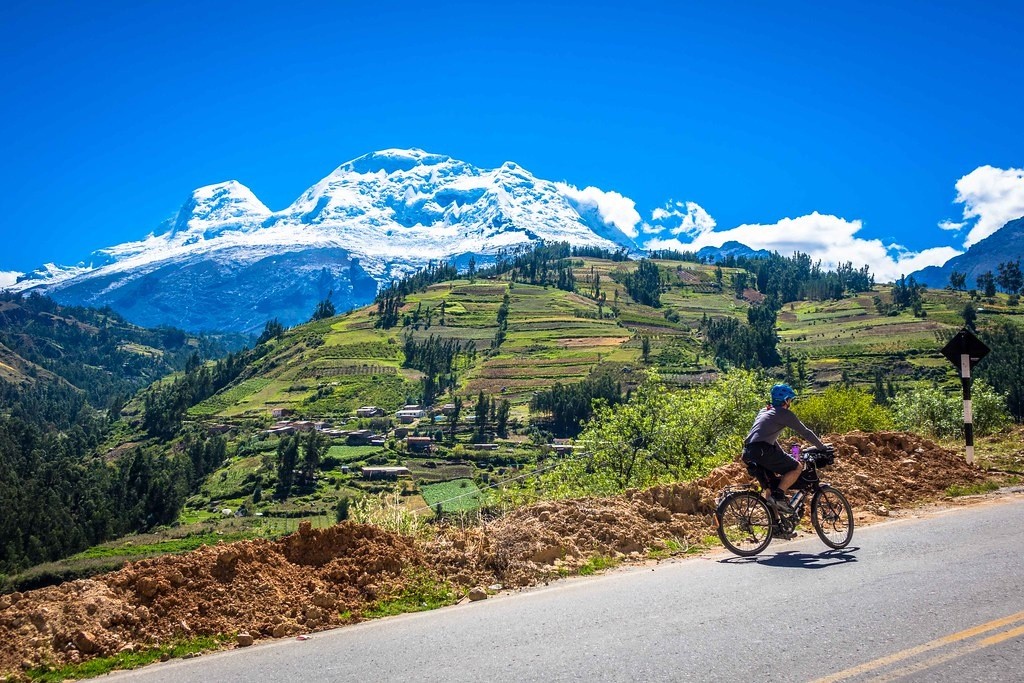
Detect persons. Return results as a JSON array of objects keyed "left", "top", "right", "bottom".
[{"left": 742, "top": 384, "right": 833, "bottom": 537}]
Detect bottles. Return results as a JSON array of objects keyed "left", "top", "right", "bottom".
[
  {"left": 790, "top": 444, "right": 801, "bottom": 461},
  {"left": 789, "top": 490, "right": 804, "bottom": 510}
]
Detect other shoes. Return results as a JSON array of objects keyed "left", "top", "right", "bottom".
[{"left": 772, "top": 528, "right": 797, "bottom": 538}]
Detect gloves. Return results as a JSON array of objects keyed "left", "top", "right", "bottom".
[{"left": 819, "top": 446, "right": 827, "bottom": 454}]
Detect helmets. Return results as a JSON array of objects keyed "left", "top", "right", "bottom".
[{"left": 771, "top": 384, "right": 798, "bottom": 405}]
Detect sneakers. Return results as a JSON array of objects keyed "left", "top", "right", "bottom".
[{"left": 766, "top": 494, "right": 792, "bottom": 513}]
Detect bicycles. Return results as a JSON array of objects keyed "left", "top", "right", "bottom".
[{"left": 712, "top": 443, "right": 855, "bottom": 557}]
